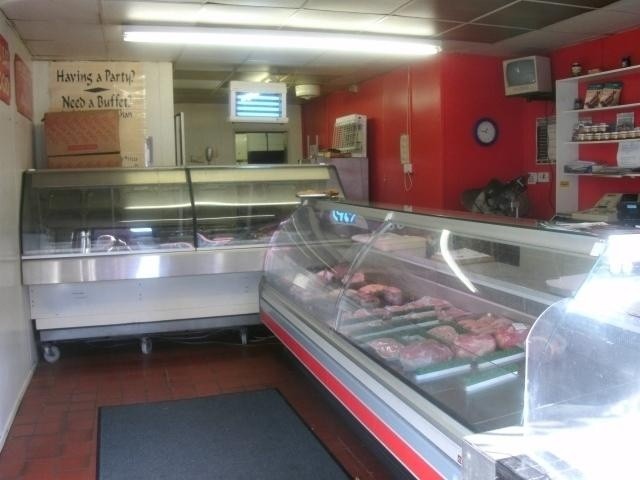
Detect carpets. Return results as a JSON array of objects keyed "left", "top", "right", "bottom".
[{"left": 96, "top": 383, "right": 354, "bottom": 480}]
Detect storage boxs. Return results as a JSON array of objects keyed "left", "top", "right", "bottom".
[{"left": 41, "top": 108, "right": 122, "bottom": 168}]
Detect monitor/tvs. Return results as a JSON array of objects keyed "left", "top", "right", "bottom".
[{"left": 503, "top": 55, "right": 552, "bottom": 96}]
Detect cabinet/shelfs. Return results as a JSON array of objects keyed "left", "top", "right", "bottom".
[{"left": 551, "top": 63, "right": 640, "bottom": 217}]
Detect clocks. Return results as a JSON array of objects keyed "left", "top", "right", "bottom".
[{"left": 473, "top": 116, "right": 498, "bottom": 146}]
